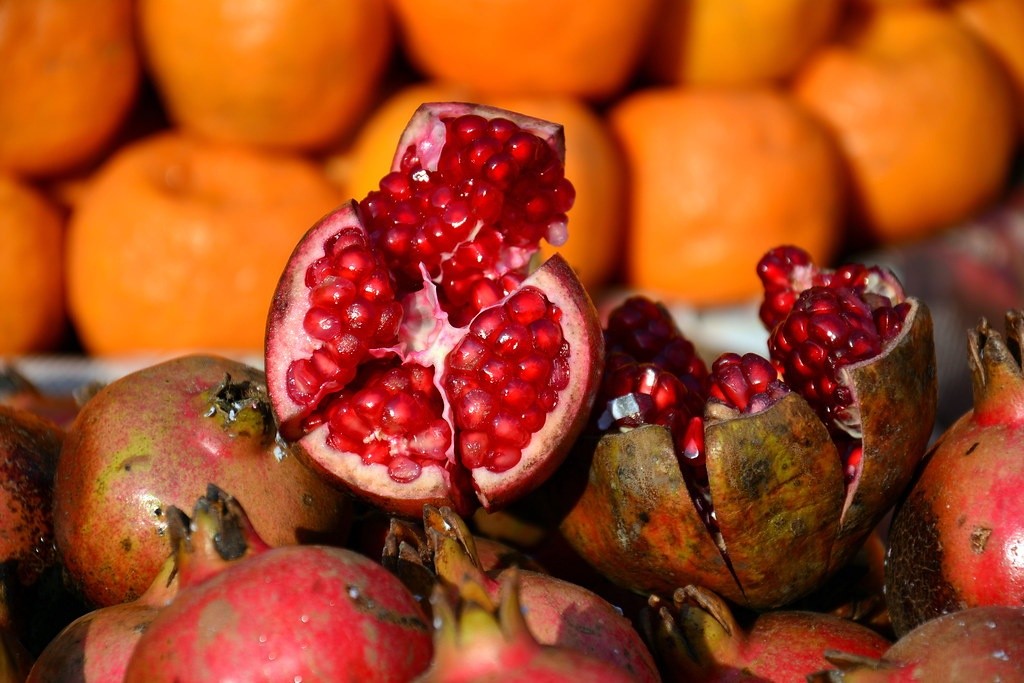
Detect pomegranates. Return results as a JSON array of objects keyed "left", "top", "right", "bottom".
[{"left": 0, "top": 103, "right": 1019, "bottom": 683}]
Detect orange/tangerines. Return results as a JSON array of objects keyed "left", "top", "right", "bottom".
[{"left": 1, "top": 0, "right": 1024, "bottom": 358}]
